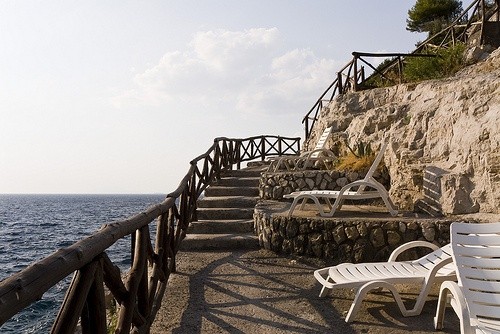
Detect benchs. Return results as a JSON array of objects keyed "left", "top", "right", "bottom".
[
  {"left": 265, "top": 125, "right": 333, "bottom": 172},
  {"left": 312, "top": 234, "right": 499, "bottom": 323},
  {"left": 434, "top": 221, "right": 500, "bottom": 334},
  {"left": 282, "top": 144, "right": 399, "bottom": 218}
]
